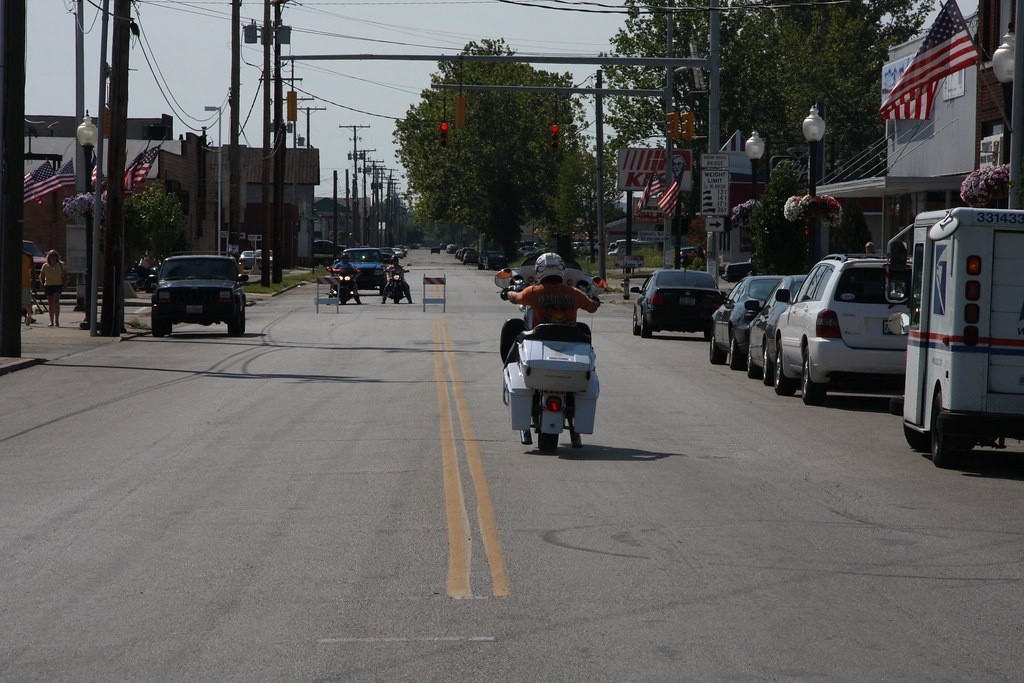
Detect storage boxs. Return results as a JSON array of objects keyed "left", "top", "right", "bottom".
[{"left": 504, "top": 339, "right": 599, "bottom": 433}]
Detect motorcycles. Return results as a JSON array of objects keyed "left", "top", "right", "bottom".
[
  {"left": 328, "top": 259, "right": 412, "bottom": 306},
  {"left": 494, "top": 252, "right": 608, "bottom": 455}
]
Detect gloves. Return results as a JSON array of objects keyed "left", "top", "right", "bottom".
[{"left": 500, "top": 289, "right": 508, "bottom": 301}]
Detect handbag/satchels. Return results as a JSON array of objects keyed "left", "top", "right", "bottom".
[{"left": 59, "top": 261, "right": 69, "bottom": 283}]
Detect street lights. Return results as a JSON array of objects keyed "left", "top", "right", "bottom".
[
  {"left": 205, "top": 106, "right": 221, "bottom": 256},
  {"left": 745, "top": 129, "right": 765, "bottom": 201},
  {"left": 992, "top": 21, "right": 1016, "bottom": 165},
  {"left": 76, "top": 109, "right": 101, "bottom": 330},
  {"left": 803, "top": 105, "right": 826, "bottom": 271}
]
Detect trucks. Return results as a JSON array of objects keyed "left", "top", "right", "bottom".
[{"left": 885, "top": 206, "right": 1023, "bottom": 470}]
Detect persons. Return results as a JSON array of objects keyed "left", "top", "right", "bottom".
[
  {"left": 500, "top": 253, "right": 600, "bottom": 331},
  {"left": 326, "top": 253, "right": 363, "bottom": 305},
  {"left": 39, "top": 250, "right": 67, "bottom": 326},
  {"left": 22, "top": 250, "right": 37, "bottom": 326},
  {"left": 381, "top": 256, "right": 413, "bottom": 304}
]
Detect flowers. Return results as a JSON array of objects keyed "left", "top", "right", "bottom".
[
  {"left": 781, "top": 193, "right": 843, "bottom": 228},
  {"left": 59, "top": 189, "right": 107, "bottom": 218},
  {"left": 727, "top": 199, "right": 757, "bottom": 228},
  {"left": 958, "top": 162, "right": 1010, "bottom": 209}
]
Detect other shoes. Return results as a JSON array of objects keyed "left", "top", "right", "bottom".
[{"left": 26, "top": 314, "right": 31, "bottom": 326}]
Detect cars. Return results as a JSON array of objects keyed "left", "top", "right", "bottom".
[
  {"left": 744, "top": 275, "right": 808, "bottom": 386},
  {"left": 630, "top": 269, "right": 726, "bottom": 342},
  {"left": 238, "top": 249, "right": 273, "bottom": 269},
  {"left": 22, "top": 240, "right": 70, "bottom": 291},
  {"left": 314, "top": 240, "right": 408, "bottom": 290},
  {"left": 431, "top": 240, "right": 756, "bottom": 282},
  {"left": 709, "top": 276, "right": 786, "bottom": 370}
]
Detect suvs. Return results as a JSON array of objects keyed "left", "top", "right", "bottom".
[
  {"left": 148, "top": 251, "right": 249, "bottom": 338},
  {"left": 774, "top": 253, "right": 913, "bottom": 406}
]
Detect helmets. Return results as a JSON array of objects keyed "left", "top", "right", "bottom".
[
  {"left": 534, "top": 252, "right": 566, "bottom": 283},
  {"left": 389, "top": 255, "right": 399, "bottom": 265},
  {"left": 340, "top": 253, "right": 350, "bottom": 260}
]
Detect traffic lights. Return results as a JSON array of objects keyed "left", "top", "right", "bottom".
[
  {"left": 666, "top": 111, "right": 694, "bottom": 141},
  {"left": 440, "top": 122, "right": 449, "bottom": 148},
  {"left": 550, "top": 124, "right": 560, "bottom": 149}
]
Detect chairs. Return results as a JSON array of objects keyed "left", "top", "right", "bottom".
[
  {"left": 696, "top": 278, "right": 709, "bottom": 288},
  {"left": 211, "top": 268, "right": 224, "bottom": 276},
  {"left": 660, "top": 277, "right": 673, "bottom": 286}
]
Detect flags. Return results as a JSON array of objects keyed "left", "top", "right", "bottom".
[
  {"left": 656, "top": 166, "right": 684, "bottom": 221},
  {"left": 634, "top": 168, "right": 664, "bottom": 216},
  {"left": 91, "top": 152, "right": 105, "bottom": 185},
  {"left": 56, "top": 159, "right": 76, "bottom": 185},
  {"left": 878, "top": 0, "right": 980, "bottom": 120},
  {"left": 125, "top": 143, "right": 161, "bottom": 190},
  {"left": 24, "top": 161, "right": 63, "bottom": 207}
]
